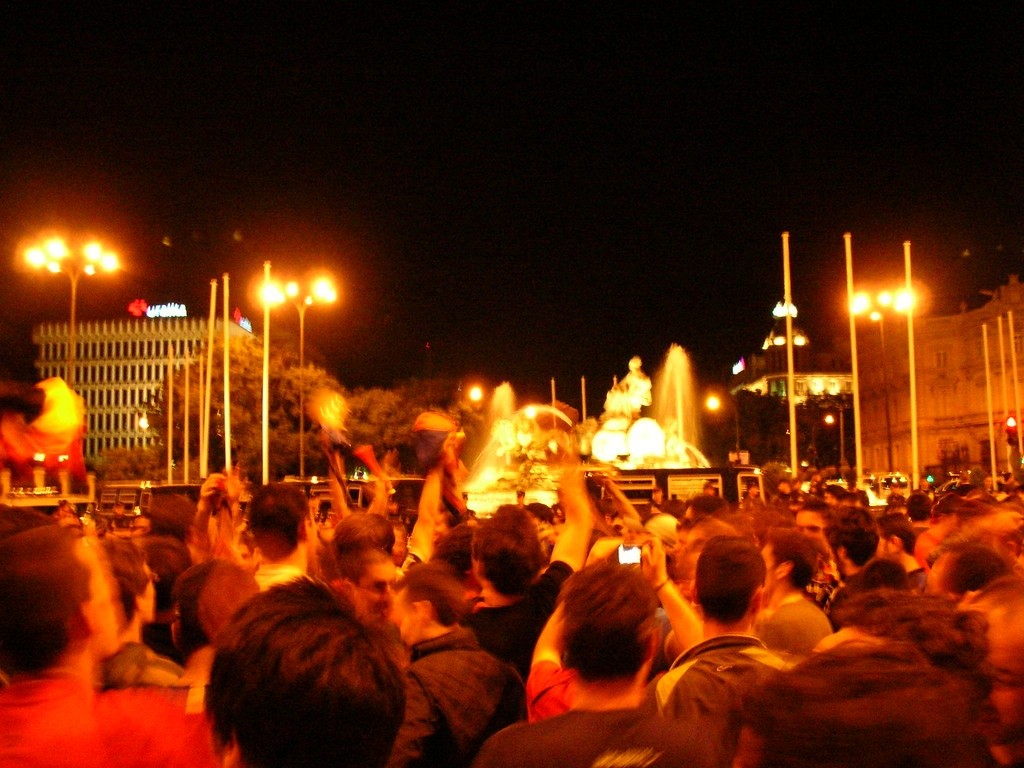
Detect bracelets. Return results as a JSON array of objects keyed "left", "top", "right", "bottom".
[{"left": 654, "top": 574, "right": 672, "bottom": 593}]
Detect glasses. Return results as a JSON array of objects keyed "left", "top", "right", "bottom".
[{"left": 146, "top": 573, "right": 161, "bottom": 583}]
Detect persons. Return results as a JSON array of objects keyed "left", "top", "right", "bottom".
[{"left": 1, "top": 430, "right": 1023, "bottom": 768}]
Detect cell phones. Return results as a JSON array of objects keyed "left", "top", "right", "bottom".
[{"left": 619, "top": 544, "right": 642, "bottom": 565}]
